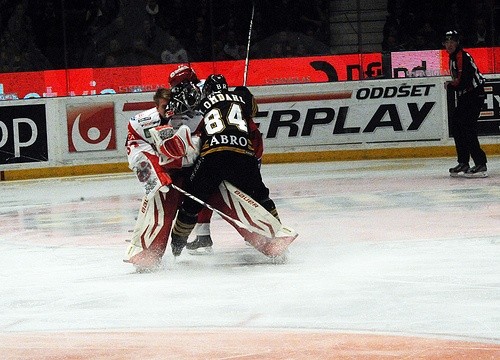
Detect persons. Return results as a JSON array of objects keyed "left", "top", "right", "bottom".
[
  {"left": 443, "top": 27, "right": 490, "bottom": 178},
  {"left": 165, "top": 64, "right": 264, "bottom": 254},
  {"left": 170, "top": 71, "right": 282, "bottom": 260},
  {"left": 126, "top": 88, "right": 298, "bottom": 273},
  {"left": 383, "top": 0, "right": 500, "bottom": 54},
  {"left": 0, "top": 0, "right": 331, "bottom": 73}
]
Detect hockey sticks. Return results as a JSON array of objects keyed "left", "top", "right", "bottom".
[
  {"left": 169, "top": 183, "right": 254, "bottom": 236},
  {"left": 242, "top": 0, "right": 257, "bottom": 88}
]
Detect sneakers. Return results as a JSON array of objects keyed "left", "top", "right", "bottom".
[
  {"left": 186, "top": 235, "right": 214, "bottom": 255},
  {"left": 448, "top": 160, "right": 471, "bottom": 177},
  {"left": 463, "top": 163, "right": 489, "bottom": 178},
  {"left": 171, "top": 236, "right": 187, "bottom": 259}
]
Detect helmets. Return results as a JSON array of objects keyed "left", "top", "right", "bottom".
[
  {"left": 202, "top": 73, "right": 229, "bottom": 97},
  {"left": 443, "top": 30, "right": 459, "bottom": 41},
  {"left": 167, "top": 64, "right": 201, "bottom": 88}
]
